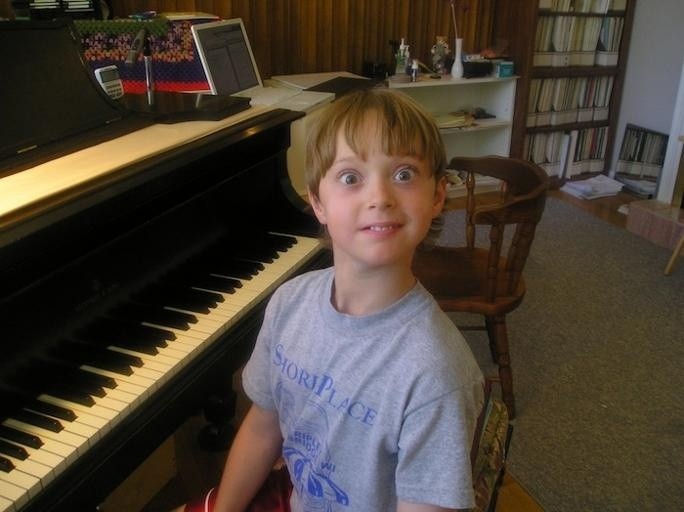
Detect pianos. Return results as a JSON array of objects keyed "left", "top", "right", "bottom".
[{"left": 1, "top": 16, "right": 333, "bottom": 512}]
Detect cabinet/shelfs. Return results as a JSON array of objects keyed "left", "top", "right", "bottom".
[
  {"left": 388, "top": 73, "right": 520, "bottom": 199},
  {"left": 490, "top": 0, "right": 637, "bottom": 191}
]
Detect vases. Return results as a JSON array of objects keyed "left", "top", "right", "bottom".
[{"left": 451, "top": 38, "right": 464, "bottom": 79}]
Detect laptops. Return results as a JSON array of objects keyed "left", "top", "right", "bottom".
[
  {"left": 271, "top": 70, "right": 372, "bottom": 91},
  {"left": 191, "top": 18, "right": 336, "bottom": 113}
]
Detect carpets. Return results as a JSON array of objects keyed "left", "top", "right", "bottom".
[{"left": 434, "top": 195, "right": 684, "bottom": 512}]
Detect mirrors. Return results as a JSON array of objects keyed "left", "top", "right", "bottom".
[{"left": 614, "top": 123, "right": 669, "bottom": 199}]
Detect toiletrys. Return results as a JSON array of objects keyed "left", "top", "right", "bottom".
[
  {"left": 406, "top": 56, "right": 413, "bottom": 75},
  {"left": 410, "top": 57, "right": 419, "bottom": 83}
]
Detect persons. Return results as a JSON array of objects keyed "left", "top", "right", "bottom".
[{"left": 172, "top": 87, "right": 487, "bottom": 512}]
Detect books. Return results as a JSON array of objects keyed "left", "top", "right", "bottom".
[
  {"left": 523, "top": 0, "right": 626, "bottom": 201},
  {"left": 621, "top": 128, "right": 665, "bottom": 195},
  {"left": 447, "top": 108, "right": 477, "bottom": 127},
  {"left": 431, "top": 111, "right": 466, "bottom": 129},
  {"left": 28, "top": 0, "right": 93, "bottom": 13}
]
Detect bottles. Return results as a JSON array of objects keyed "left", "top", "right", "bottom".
[{"left": 411, "top": 60, "right": 418, "bottom": 82}]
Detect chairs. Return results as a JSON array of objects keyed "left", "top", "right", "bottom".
[{"left": 411, "top": 154, "right": 549, "bottom": 421}]
